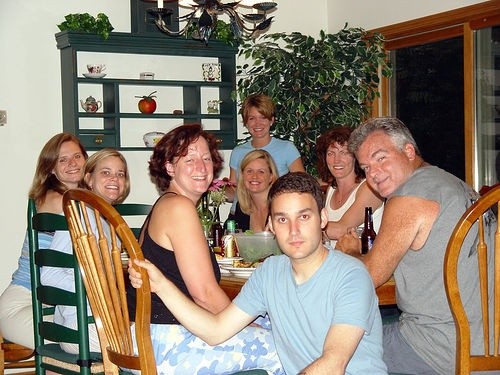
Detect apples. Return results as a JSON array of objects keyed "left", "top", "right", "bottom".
[{"left": 138, "top": 96, "right": 156, "bottom": 113}]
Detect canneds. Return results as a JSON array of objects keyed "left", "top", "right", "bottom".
[{"left": 221, "top": 235, "right": 237, "bottom": 257}]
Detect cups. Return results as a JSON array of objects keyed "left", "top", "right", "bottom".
[{"left": 87, "top": 64, "right": 105, "bottom": 74}]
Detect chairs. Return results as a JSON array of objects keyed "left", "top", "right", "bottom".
[
  {"left": 0, "top": 187, "right": 268, "bottom": 375},
  {"left": 444, "top": 186, "right": 500, "bottom": 375}
]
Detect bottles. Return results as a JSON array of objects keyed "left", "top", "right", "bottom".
[
  {"left": 202, "top": 192, "right": 214, "bottom": 238},
  {"left": 227, "top": 220, "right": 237, "bottom": 236},
  {"left": 361, "top": 207, "right": 377, "bottom": 255},
  {"left": 212, "top": 202, "right": 223, "bottom": 247}
]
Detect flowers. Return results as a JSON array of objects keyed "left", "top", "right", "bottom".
[{"left": 198, "top": 177, "right": 237, "bottom": 228}]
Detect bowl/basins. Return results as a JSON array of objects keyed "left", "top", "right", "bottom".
[
  {"left": 140, "top": 73, "right": 154, "bottom": 79},
  {"left": 234, "top": 231, "right": 283, "bottom": 263},
  {"left": 143, "top": 132, "right": 166, "bottom": 147}
]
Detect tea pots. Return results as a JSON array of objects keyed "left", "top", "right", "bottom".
[{"left": 80, "top": 96, "right": 102, "bottom": 113}]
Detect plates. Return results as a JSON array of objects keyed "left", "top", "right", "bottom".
[
  {"left": 217, "top": 257, "right": 243, "bottom": 264},
  {"left": 221, "top": 265, "right": 256, "bottom": 277},
  {"left": 120, "top": 253, "right": 130, "bottom": 261},
  {"left": 83, "top": 73, "right": 107, "bottom": 79}
]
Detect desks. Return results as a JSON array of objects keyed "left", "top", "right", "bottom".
[{"left": 123, "top": 264, "right": 396, "bottom": 306}]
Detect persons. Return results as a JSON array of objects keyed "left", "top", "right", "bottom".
[
  {"left": 129, "top": 172, "right": 388, "bottom": 375},
  {"left": 42, "top": 148, "right": 130, "bottom": 354},
  {"left": 223, "top": 96, "right": 306, "bottom": 228},
  {"left": 0, "top": 132, "right": 89, "bottom": 350},
  {"left": 131, "top": 123, "right": 285, "bottom": 375},
  {"left": 348, "top": 117, "right": 500, "bottom": 375},
  {"left": 315, "top": 126, "right": 385, "bottom": 249},
  {"left": 223, "top": 149, "right": 279, "bottom": 233}
]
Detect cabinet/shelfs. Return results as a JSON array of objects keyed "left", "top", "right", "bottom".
[{"left": 55, "top": 31, "right": 239, "bottom": 151}]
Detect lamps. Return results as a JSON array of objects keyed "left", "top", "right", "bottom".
[{"left": 145, "top": 0, "right": 278, "bottom": 44}]
